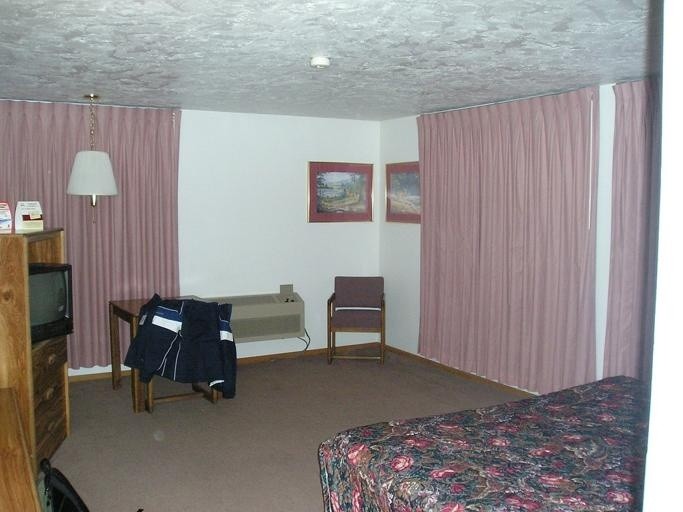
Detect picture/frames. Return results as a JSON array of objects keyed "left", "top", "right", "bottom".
[
  {"left": 384, "top": 159, "right": 423, "bottom": 226},
  {"left": 304, "top": 159, "right": 374, "bottom": 224}
]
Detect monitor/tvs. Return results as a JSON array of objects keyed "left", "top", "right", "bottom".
[{"left": 29, "top": 263, "right": 73, "bottom": 343}]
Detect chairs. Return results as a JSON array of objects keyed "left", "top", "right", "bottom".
[
  {"left": 122, "top": 294, "right": 236, "bottom": 414},
  {"left": 325, "top": 273, "right": 387, "bottom": 367}
]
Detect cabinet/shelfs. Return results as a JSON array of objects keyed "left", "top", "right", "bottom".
[{"left": 0, "top": 227, "right": 72, "bottom": 480}]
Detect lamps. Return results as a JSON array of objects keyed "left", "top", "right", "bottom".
[{"left": 62, "top": 86, "right": 119, "bottom": 208}]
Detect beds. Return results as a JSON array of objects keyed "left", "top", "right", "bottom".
[{"left": 316, "top": 373, "right": 649, "bottom": 512}]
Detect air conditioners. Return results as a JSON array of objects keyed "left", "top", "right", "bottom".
[{"left": 194, "top": 290, "right": 305, "bottom": 343}]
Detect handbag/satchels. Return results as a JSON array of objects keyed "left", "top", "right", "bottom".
[{"left": 40, "top": 458, "right": 88, "bottom": 512}]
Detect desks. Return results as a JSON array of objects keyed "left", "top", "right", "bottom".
[
  {"left": 0, "top": 384, "right": 43, "bottom": 512},
  {"left": 106, "top": 293, "right": 176, "bottom": 414}
]
